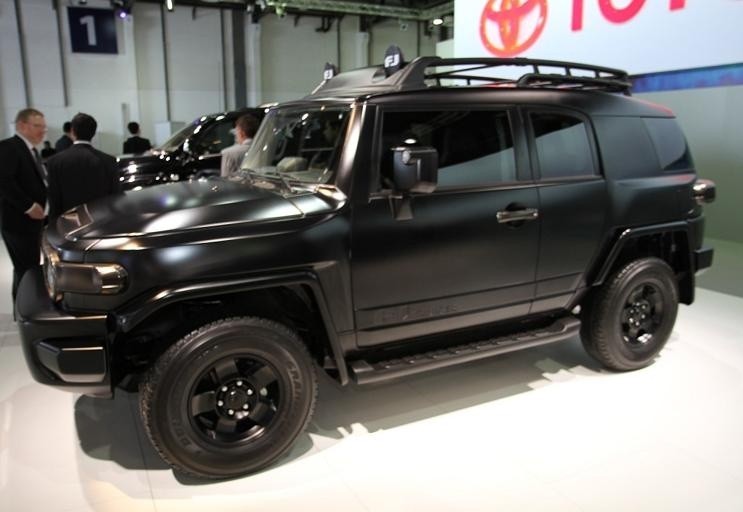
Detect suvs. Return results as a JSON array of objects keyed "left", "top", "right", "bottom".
[
  {"left": 12, "top": 52, "right": 717, "bottom": 490},
  {"left": 101, "top": 103, "right": 274, "bottom": 197}
]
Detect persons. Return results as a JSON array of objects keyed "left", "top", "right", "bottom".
[
  {"left": 123, "top": 122, "right": 151, "bottom": 155},
  {"left": 217, "top": 112, "right": 258, "bottom": 178},
  {"left": 48, "top": 116, "right": 121, "bottom": 229},
  {"left": 53, "top": 121, "right": 73, "bottom": 153},
  {"left": 0, "top": 109, "right": 46, "bottom": 325},
  {"left": 40, "top": 141, "right": 56, "bottom": 156}
]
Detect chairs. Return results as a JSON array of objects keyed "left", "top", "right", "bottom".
[{"left": 424, "top": 127, "right": 487, "bottom": 182}]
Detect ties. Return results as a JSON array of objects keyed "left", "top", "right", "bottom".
[{"left": 33, "top": 148, "right": 46, "bottom": 180}]
